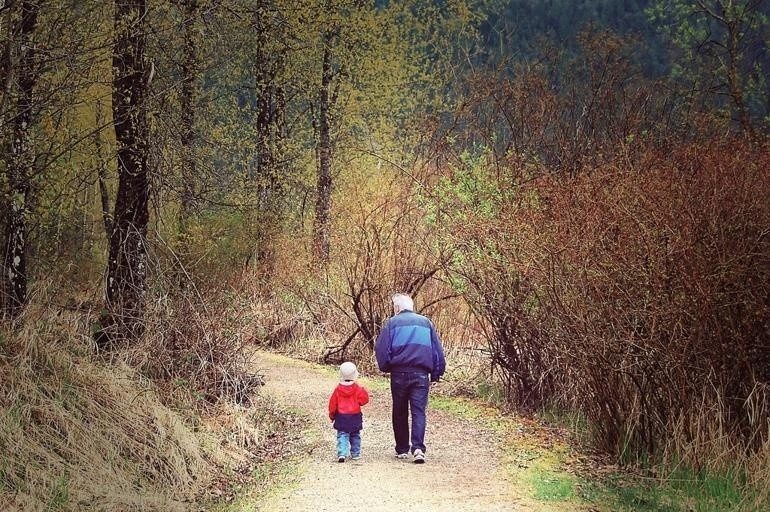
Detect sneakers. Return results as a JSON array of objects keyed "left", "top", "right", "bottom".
[
  {"left": 395, "top": 453, "right": 407, "bottom": 458},
  {"left": 352, "top": 457, "right": 361, "bottom": 460},
  {"left": 413, "top": 449, "right": 425, "bottom": 463},
  {"left": 338, "top": 455, "right": 345, "bottom": 463}
]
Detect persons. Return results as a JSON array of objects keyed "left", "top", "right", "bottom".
[
  {"left": 374, "top": 294, "right": 445, "bottom": 463},
  {"left": 328, "top": 362, "right": 369, "bottom": 462}
]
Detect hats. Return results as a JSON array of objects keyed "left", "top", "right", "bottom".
[{"left": 338, "top": 362, "right": 358, "bottom": 381}]
